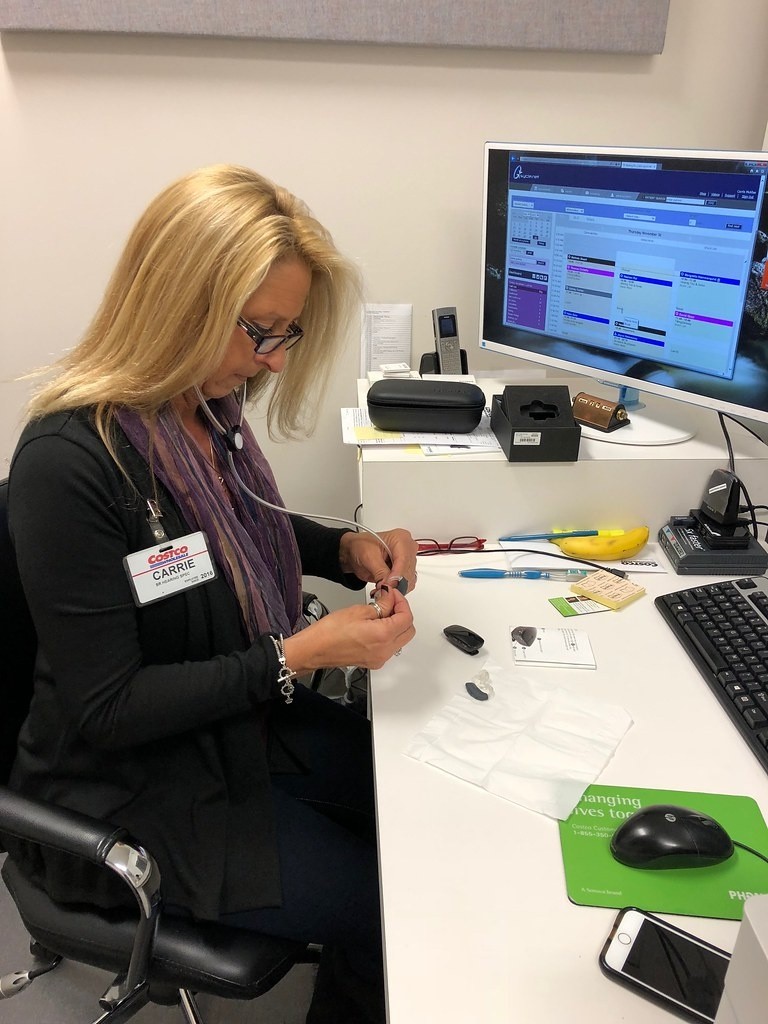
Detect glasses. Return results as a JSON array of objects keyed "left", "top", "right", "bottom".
[
  {"left": 414, "top": 536, "right": 487, "bottom": 556},
  {"left": 237, "top": 315, "right": 305, "bottom": 354}
]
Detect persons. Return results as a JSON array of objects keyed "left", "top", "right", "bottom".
[
  {"left": 0, "top": 161, "right": 420, "bottom": 1024},
  {"left": 566, "top": 596, "right": 588, "bottom": 603}
]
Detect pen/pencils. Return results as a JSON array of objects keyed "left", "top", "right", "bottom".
[{"left": 499, "top": 531, "right": 599, "bottom": 542}]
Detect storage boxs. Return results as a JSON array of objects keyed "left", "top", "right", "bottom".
[
  {"left": 367, "top": 379, "right": 486, "bottom": 434},
  {"left": 490, "top": 394, "right": 582, "bottom": 463}
]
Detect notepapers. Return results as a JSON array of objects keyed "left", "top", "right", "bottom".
[{"left": 570, "top": 569, "right": 646, "bottom": 610}]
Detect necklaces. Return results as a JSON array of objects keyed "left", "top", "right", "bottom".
[{"left": 207, "top": 428, "right": 224, "bottom": 484}]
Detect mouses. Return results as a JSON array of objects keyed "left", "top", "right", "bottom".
[{"left": 610, "top": 804, "right": 735, "bottom": 870}]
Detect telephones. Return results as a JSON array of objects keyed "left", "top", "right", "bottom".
[{"left": 420, "top": 306, "right": 470, "bottom": 375}]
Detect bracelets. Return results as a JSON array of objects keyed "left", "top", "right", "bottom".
[{"left": 271, "top": 634, "right": 296, "bottom": 705}]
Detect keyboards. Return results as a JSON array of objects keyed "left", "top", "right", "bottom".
[{"left": 654, "top": 577, "right": 768, "bottom": 776}]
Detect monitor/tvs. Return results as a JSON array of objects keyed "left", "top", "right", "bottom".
[{"left": 476, "top": 140, "right": 768, "bottom": 445}]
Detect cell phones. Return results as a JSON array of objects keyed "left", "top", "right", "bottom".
[{"left": 598, "top": 907, "right": 731, "bottom": 1024}]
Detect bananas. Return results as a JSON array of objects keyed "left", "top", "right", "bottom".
[{"left": 548, "top": 526, "right": 649, "bottom": 561}]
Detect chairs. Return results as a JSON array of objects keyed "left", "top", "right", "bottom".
[{"left": 0, "top": 480, "right": 322, "bottom": 1024}]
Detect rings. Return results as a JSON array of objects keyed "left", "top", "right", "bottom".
[
  {"left": 395, "top": 648, "right": 402, "bottom": 656},
  {"left": 370, "top": 603, "right": 383, "bottom": 618},
  {"left": 415, "top": 573, "right": 417, "bottom": 576}
]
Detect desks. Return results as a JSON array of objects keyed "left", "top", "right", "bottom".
[
  {"left": 365, "top": 511, "right": 768, "bottom": 1024},
  {"left": 353, "top": 362, "right": 768, "bottom": 543}
]
